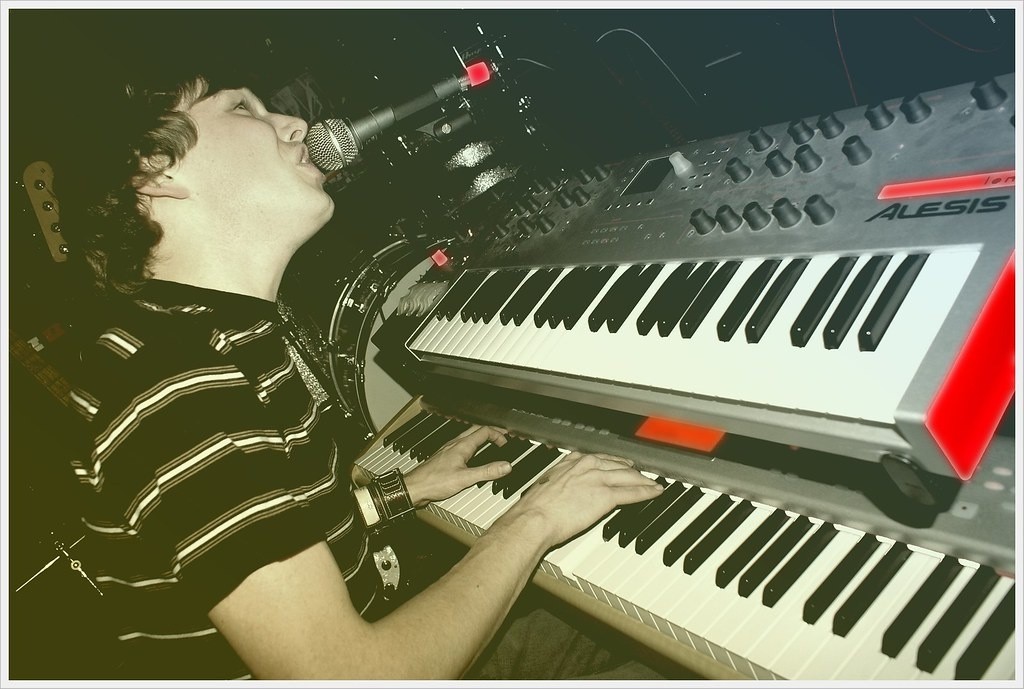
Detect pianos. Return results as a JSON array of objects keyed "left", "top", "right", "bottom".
[
  {"left": 347, "top": 386, "right": 1015, "bottom": 680},
  {"left": 369, "top": 69, "right": 1014, "bottom": 480}
]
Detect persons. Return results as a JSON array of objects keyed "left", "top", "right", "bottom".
[{"left": 9, "top": 50, "right": 665, "bottom": 681}]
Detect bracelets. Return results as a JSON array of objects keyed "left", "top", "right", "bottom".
[{"left": 355, "top": 470, "right": 415, "bottom": 536}]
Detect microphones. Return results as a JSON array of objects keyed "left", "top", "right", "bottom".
[{"left": 308, "top": 63, "right": 494, "bottom": 171}]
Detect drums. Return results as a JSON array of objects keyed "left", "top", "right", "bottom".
[{"left": 327, "top": 231, "right": 457, "bottom": 439}]
